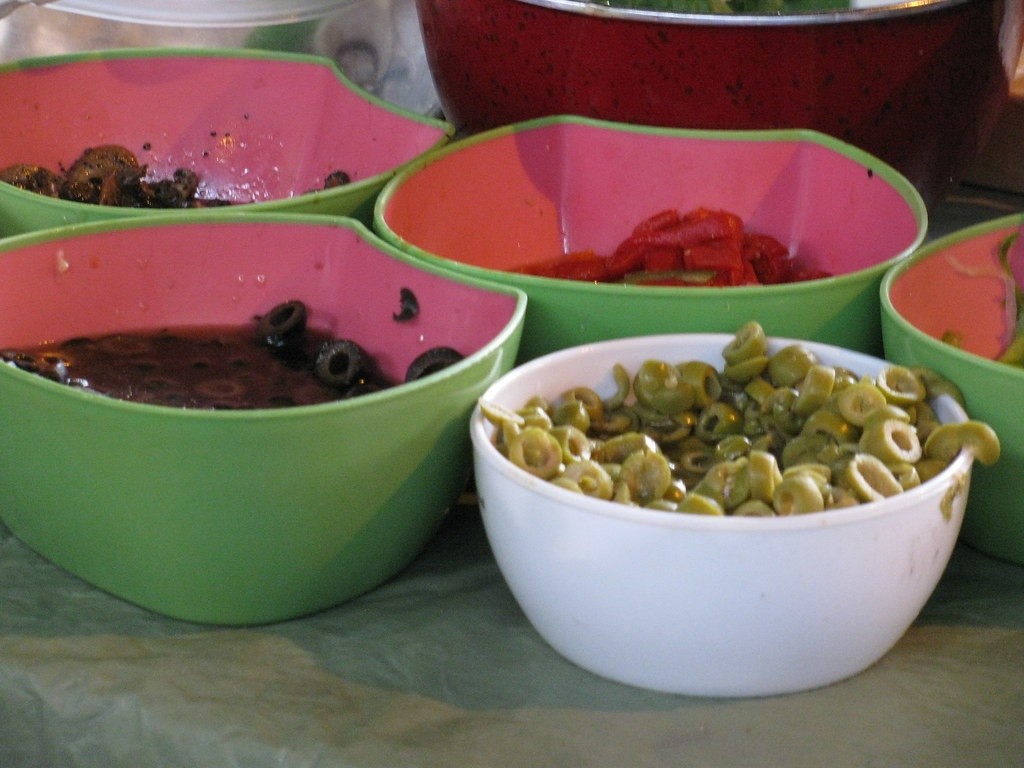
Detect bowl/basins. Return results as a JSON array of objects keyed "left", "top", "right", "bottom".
[{"left": 0, "top": 0, "right": 1024, "bottom": 697}]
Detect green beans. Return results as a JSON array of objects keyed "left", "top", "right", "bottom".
[{"left": 479, "top": 320, "right": 999, "bottom": 516}]
